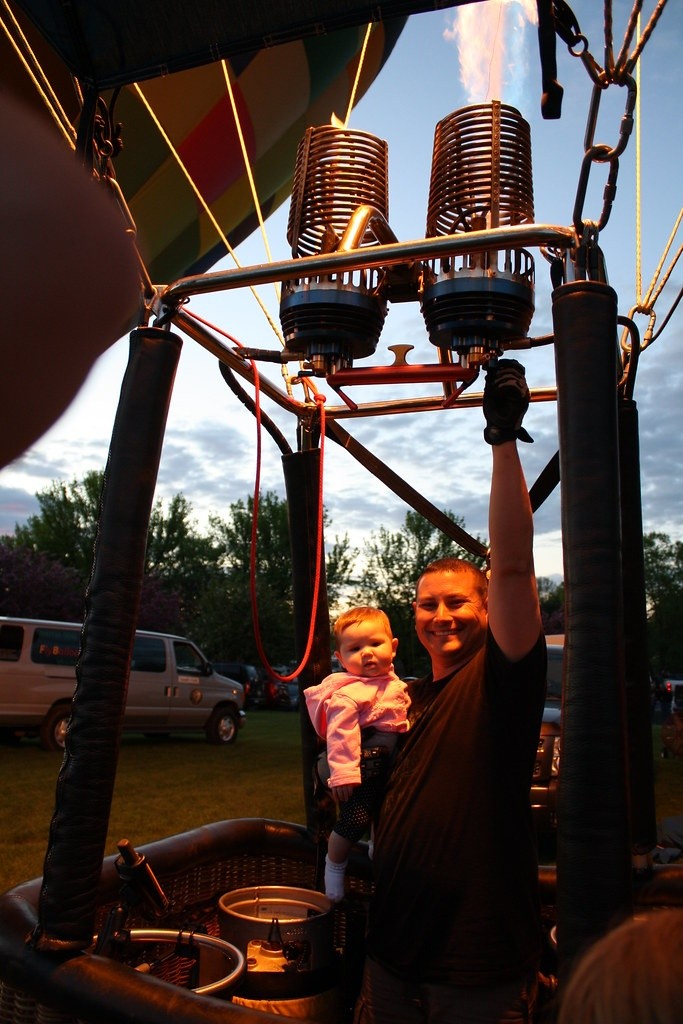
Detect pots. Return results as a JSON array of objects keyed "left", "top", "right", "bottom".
[
  {"left": 92, "top": 928, "right": 247, "bottom": 995},
  {"left": 219, "top": 884, "right": 333, "bottom": 952}
]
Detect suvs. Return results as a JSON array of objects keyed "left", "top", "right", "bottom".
[
  {"left": 181, "top": 660, "right": 299, "bottom": 711},
  {"left": 531, "top": 644, "right": 563, "bottom": 865}
]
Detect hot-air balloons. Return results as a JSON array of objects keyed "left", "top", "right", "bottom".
[{"left": 0, "top": 0, "right": 683, "bottom": 1024}]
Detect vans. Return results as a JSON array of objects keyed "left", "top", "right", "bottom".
[{"left": 0, "top": 616, "right": 244, "bottom": 754}]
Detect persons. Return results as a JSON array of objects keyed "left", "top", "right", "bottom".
[
  {"left": 312, "top": 358, "right": 548, "bottom": 1024},
  {"left": 303, "top": 607, "right": 411, "bottom": 901},
  {"left": 557, "top": 909, "right": 683, "bottom": 1024}
]
